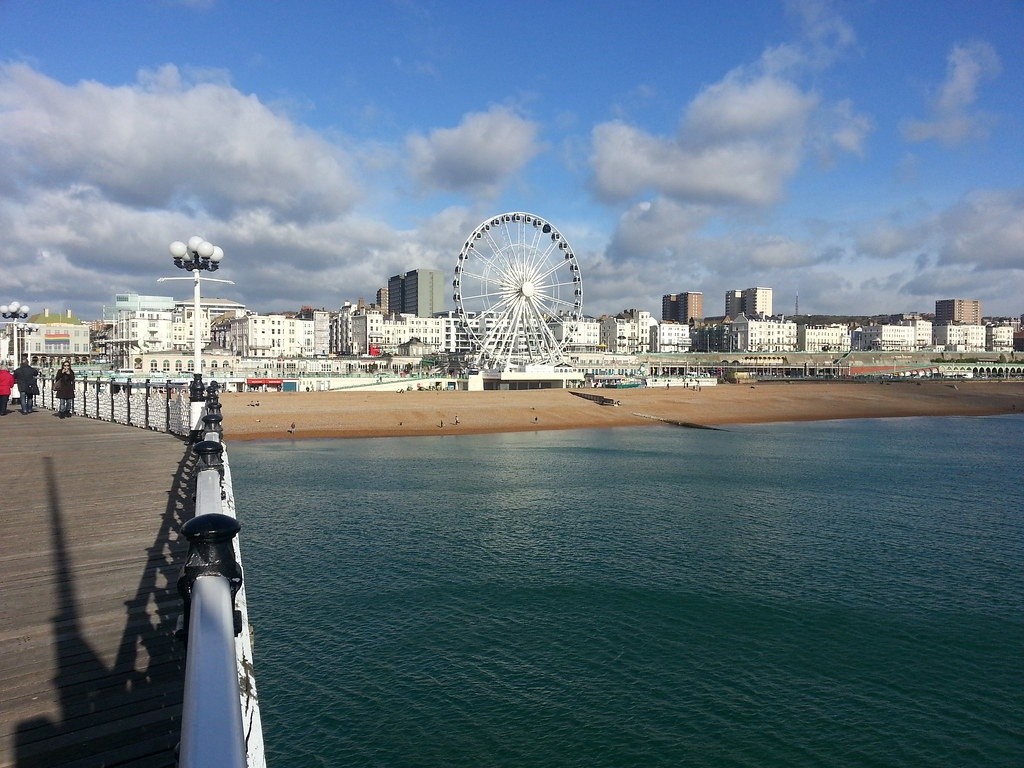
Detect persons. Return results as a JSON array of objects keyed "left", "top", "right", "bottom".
[
  {"left": 55, "top": 360, "right": 75, "bottom": 418},
  {"left": 0, "top": 364, "right": 15, "bottom": 416},
  {"left": 455, "top": 416, "right": 460, "bottom": 425},
  {"left": 396, "top": 385, "right": 445, "bottom": 393},
  {"left": 534, "top": 416, "right": 539, "bottom": 424},
  {"left": 667, "top": 377, "right": 702, "bottom": 393},
  {"left": 14, "top": 358, "right": 38, "bottom": 415},
  {"left": 250, "top": 400, "right": 260, "bottom": 407},
  {"left": 291, "top": 422, "right": 296, "bottom": 434}
]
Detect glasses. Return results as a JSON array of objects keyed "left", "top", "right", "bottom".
[{"left": 64, "top": 365, "right": 69, "bottom": 367}]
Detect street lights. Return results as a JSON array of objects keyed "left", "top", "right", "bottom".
[
  {"left": 0, "top": 302, "right": 30, "bottom": 384},
  {"left": 157, "top": 236, "right": 236, "bottom": 445}
]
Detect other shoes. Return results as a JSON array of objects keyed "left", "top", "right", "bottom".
[
  {"left": 65, "top": 411, "right": 71, "bottom": 417},
  {"left": 59, "top": 411, "right": 66, "bottom": 419}
]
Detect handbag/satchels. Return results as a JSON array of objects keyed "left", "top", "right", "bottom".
[
  {"left": 25, "top": 383, "right": 39, "bottom": 395},
  {"left": 53, "top": 379, "right": 62, "bottom": 390}
]
